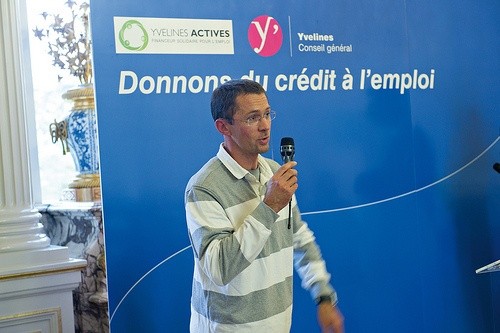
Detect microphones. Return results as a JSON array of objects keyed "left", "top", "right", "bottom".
[{"left": 280, "top": 137, "right": 295, "bottom": 201}]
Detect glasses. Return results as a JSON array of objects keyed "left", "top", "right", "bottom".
[{"left": 225, "top": 111, "right": 276, "bottom": 127}]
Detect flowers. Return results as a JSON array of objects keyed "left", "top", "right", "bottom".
[{"left": 32, "top": 0, "right": 93, "bottom": 85}]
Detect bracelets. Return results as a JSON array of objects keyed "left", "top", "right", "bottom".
[{"left": 314, "top": 292, "right": 331, "bottom": 307}]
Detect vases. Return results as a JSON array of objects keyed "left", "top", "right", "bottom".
[{"left": 50, "top": 86, "right": 102, "bottom": 174}]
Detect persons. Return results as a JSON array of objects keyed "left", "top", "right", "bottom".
[{"left": 184, "top": 80, "right": 343, "bottom": 333}]
